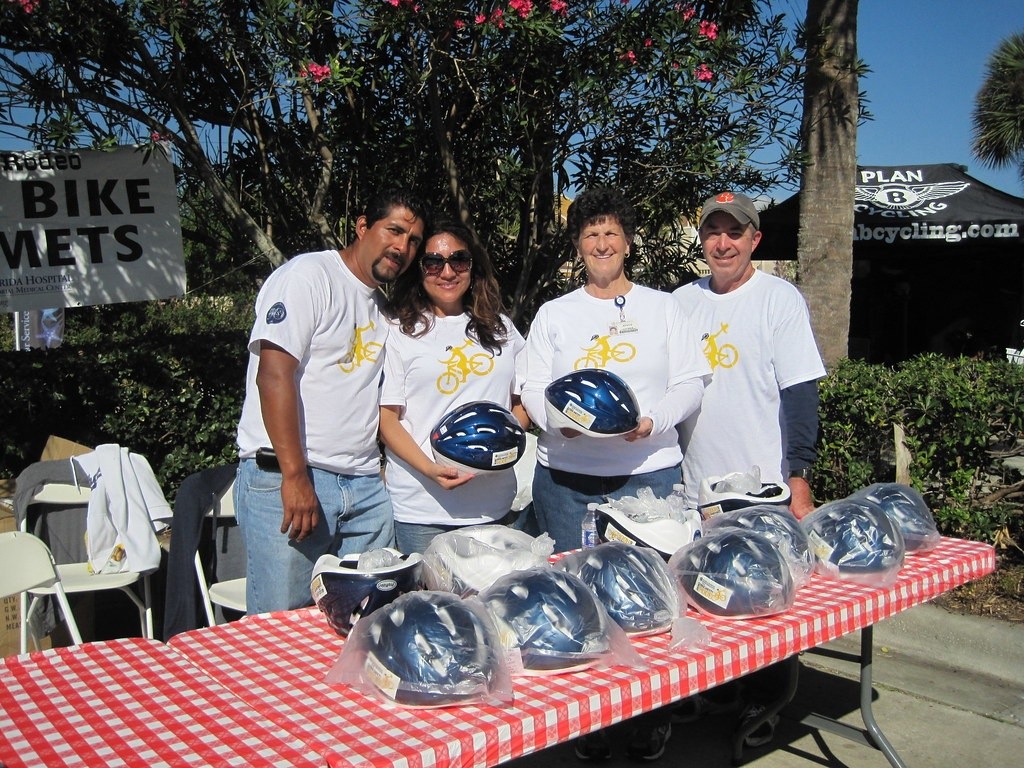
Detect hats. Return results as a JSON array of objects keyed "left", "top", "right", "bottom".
[{"left": 700, "top": 192, "right": 760, "bottom": 231}]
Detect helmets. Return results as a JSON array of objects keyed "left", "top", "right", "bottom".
[
  {"left": 594, "top": 494, "right": 704, "bottom": 563},
  {"left": 715, "top": 506, "right": 811, "bottom": 574},
  {"left": 574, "top": 541, "right": 680, "bottom": 638},
  {"left": 545, "top": 368, "right": 640, "bottom": 438},
  {"left": 677, "top": 530, "right": 794, "bottom": 620},
  {"left": 802, "top": 499, "right": 905, "bottom": 574},
  {"left": 359, "top": 590, "right": 501, "bottom": 707},
  {"left": 482, "top": 565, "right": 609, "bottom": 675},
  {"left": 432, "top": 524, "right": 545, "bottom": 603},
  {"left": 864, "top": 484, "right": 927, "bottom": 552},
  {"left": 430, "top": 401, "right": 526, "bottom": 477},
  {"left": 309, "top": 546, "right": 423, "bottom": 642},
  {"left": 697, "top": 475, "right": 793, "bottom": 517}
]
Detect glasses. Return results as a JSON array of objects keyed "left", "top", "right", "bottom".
[{"left": 419, "top": 249, "right": 473, "bottom": 274}]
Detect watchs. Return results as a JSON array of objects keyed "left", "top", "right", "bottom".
[{"left": 790, "top": 468, "right": 813, "bottom": 483}]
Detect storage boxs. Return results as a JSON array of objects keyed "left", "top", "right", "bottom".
[{"left": 0, "top": 436, "right": 142, "bottom": 655}]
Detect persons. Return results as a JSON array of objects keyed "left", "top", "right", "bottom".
[
  {"left": 231, "top": 186, "right": 435, "bottom": 616},
  {"left": 379, "top": 221, "right": 532, "bottom": 591},
  {"left": 520, "top": 186, "right": 713, "bottom": 760},
  {"left": 671, "top": 191, "right": 827, "bottom": 747}
]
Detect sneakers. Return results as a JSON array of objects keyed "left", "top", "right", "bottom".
[
  {"left": 624, "top": 720, "right": 672, "bottom": 761},
  {"left": 731, "top": 702, "right": 780, "bottom": 746},
  {"left": 574, "top": 730, "right": 612, "bottom": 760}
]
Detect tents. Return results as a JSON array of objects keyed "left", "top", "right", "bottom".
[{"left": 750, "top": 163, "right": 1024, "bottom": 363}]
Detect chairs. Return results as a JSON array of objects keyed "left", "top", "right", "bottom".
[{"left": 0, "top": 432, "right": 249, "bottom": 655}]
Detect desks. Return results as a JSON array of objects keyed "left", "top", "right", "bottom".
[{"left": 0, "top": 533, "right": 993, "bottom": 768}]
